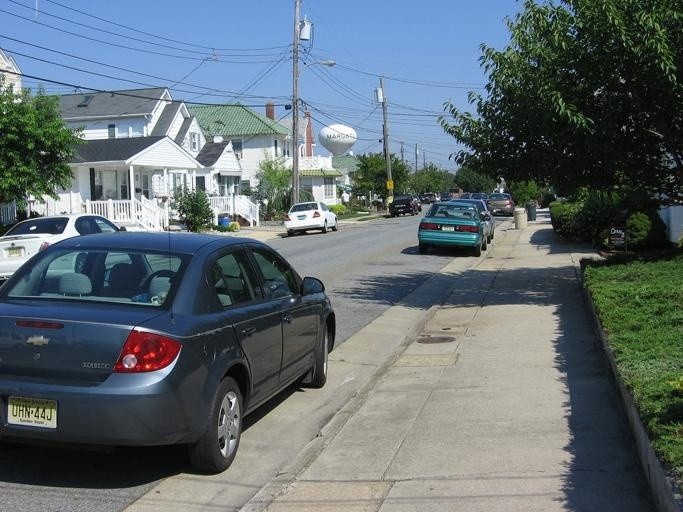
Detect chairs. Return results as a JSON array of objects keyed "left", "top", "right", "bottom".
[
  {"left": 101, "top": 263, "right": 145, "bottom": 297},
  {"left": 150, "top": 273, "right": 172, "bottom": 302},
  {"left": 59, "top": 273, "right": 93, "bottom": 299},
  {"left": 209, "top": 262, "right": 234, "bottom": 308}
]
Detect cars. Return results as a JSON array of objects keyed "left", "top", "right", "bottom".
[
  {"left": 283, "top": 201, "right": 339, "bottom": 236},
  {"left": 387, "top": 190, "right": 516, "bottom": 257},
  {"left": 0, "top": 212, "right": 131, "bottom": 290},
  {"left": 0, "top": 227, "right": 337, "bottom": 478}
]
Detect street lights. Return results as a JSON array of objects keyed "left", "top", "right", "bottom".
[{"left": 289, "top": 59, "right": 337, "bottom": 205}]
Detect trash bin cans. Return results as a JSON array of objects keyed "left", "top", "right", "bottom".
[
  {"left": 220, "top": 217, "right": 230, "bottom": 228},
  {"left": 513, "top": 207, "right": 528, "bottom": 229},
  {"left": 525, "top": 202, "right": 536, "bottom": 221}
]
